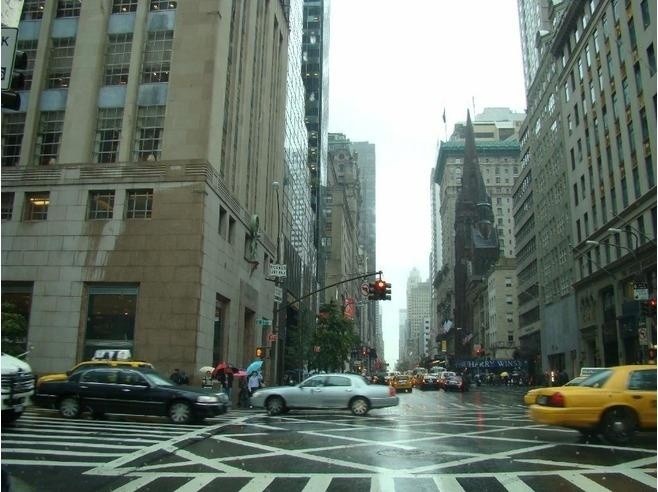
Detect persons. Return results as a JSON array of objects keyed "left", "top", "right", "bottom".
[
  {"left": 169, "top": 369, "right": 298, "bottom": 410},
  {"left": 460, "top": 368, "right": 571, "bottom": 390}
]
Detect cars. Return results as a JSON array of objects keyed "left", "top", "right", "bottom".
[
  {"left": 247, "top": 373, "right": 399, "bottom": 416},
  {"left": 1, "top": 349, "right": 229, "bottom": 431},
  {"left": 520, "top": 364, "right": 656, "bottom": 448},
  {"left": 365, "top": 365, "right": 469, "bottom": 394}
]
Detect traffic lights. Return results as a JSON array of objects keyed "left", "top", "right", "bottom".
[
  {"left": 375, "top": 281, "right": 385, "bottom": 300},
  {"left": 381, "top": 282, "right": 391, "bottom": 300},
  {"left": 646, "top": 348, "right": 655, "bottom": 359},
  {"left": 647, "top": 298, "right": 656, "bottom": 315},
  {"left": 368, "top": 281, "right": 378, "bottom": 300},
  {"left": 255, "top": 348, "right": 265, "bottom": 359}
]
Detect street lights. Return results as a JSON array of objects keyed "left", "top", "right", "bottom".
[
  {"left": 271, "top": 180, "right": 284, "bottom": 386},
  {"left": 583, "top": 227, "right": 651, "bottom": 366}
]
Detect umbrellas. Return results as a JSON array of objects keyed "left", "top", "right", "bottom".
[
  {"left": 247, "top": 360, "right": 264, "bottom": 375},
  {"left": 214, "top": 361, "right": 233, "bottom": 373},
  {"left": 199, "top": 364, "right": 214, "bottom": 374},
  {"left": 308, "top": 368, "right": 325, "bottom": 374}
]
